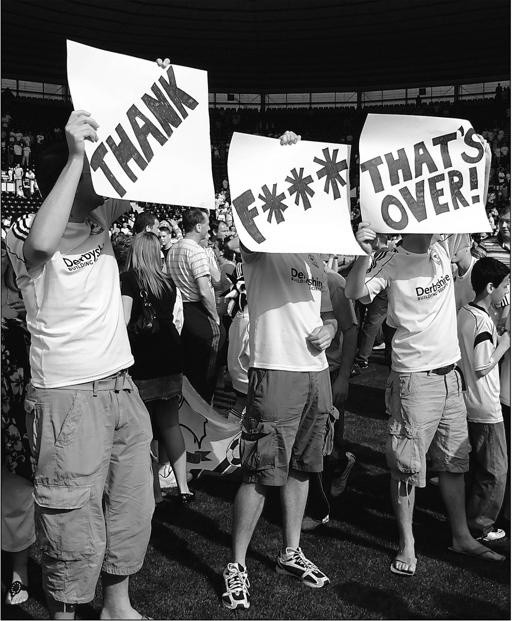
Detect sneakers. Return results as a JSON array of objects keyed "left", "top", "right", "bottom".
[
  {"left": 274, "top": 543, "right": 332, "bottom": 590},
  {"left": 477, "top": 527, "right": 506, "bottom": 545},
  {"left": 219, "top": 560, "right": 253, "bottom": 610},
  {"left": 328, "top": 450, "right": 357, "bottom": 498},
  {"left": 300, "top": 511, "right": 331, "bottom": 533}
]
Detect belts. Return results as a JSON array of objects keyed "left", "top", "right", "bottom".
[
  {"left": 421, "top": 362, "right": 469, "bottom": 395},
  {"left": 56, "top": 372, "right": 139, "bottom": 392}
]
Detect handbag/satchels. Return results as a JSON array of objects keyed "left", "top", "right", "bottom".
[{"left": 126, "top": 279, "right": 162, "bottom": 345}]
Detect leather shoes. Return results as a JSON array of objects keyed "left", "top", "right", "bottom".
[
  {"left": 156, "top": 497, "right": 173, "bottom": 508},
  {"left": 180, "top": 489, "right": 197, "bottom": 504}
]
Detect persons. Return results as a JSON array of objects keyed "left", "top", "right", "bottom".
[
  {"left": 1, "top": 215, "right": 12, "bottom": 234},
  {"left": 428, "top": 234, "right": 511, "bottom": 488},
  {"left": 5, "top": 57, "right": 172, "bottom": 621},
  {"left": 212, "top": 238, "right": 236, "bottom": 393},
  {"left": 456, "top": 257, "right": 511, "bottom": 542},
  {"left": 0, "top": 109, "right": 36, "bottom": 197},
  {"left": 351, "top": 235, "right": 400, "bottom": 376},
  {"left": 344, "top": 136, "right": 506, "bottom": 576},
  {"left": 300, "top": 263, "right": 357, "bottom": 532},
  {"left": 500, "top": 310, "right": 511, "bottom": 526},
  {"left": 167, "top": 211, "right": 228, "bottom": 409},
  {"left": 1, "top": 317, "right": 35, "bottom": 604},
  {"left": 471, "top": 85, "right": 510, "bottom": 267},
  {"left": 1, "top": 228, "right": 26, "bottom": 317},
  {"left": 110, "top": 179, "right": 237, "bottom": 265},
  {"left": 221, "top": 274, "right": 250, "bottom": 431},
  {"left": 219, "top": 130, "right": 338, "bottom": 610},
  {"left": 119, "top": 232, "right": 195, "bottom": 505}
]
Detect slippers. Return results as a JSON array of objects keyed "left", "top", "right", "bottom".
[
  {"left": 390, "top": 555, "right": 418, "bottom": 576},
  {"left": 447, "top": 545, "right": 507, "bottom": 563},
  {"left": 3, "top": 580, "right": 32, "bottom": 606}
]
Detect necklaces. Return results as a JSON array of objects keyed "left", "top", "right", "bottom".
[{"left": 0, "top": 287, "right": 10, "bottom": 307}]
